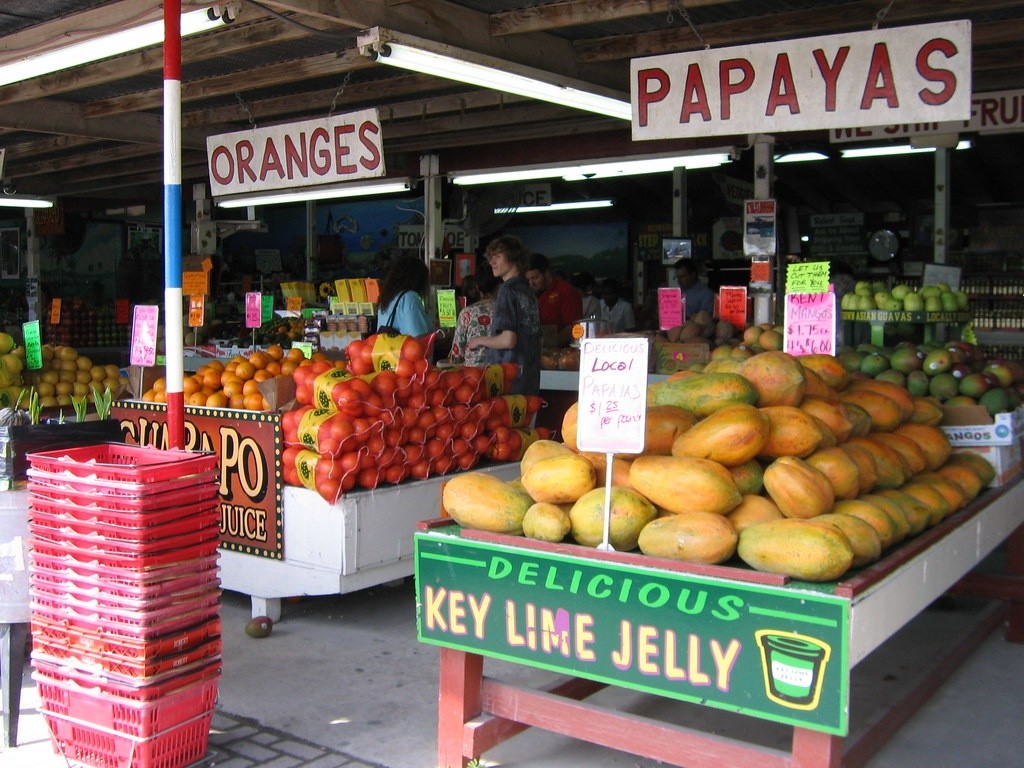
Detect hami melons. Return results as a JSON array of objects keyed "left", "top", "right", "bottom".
[{"left": 156, "top": 314, "right": 222, "bottom": 352}]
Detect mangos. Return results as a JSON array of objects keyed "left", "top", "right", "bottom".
[
  {"left": 691, "top": 323, "right": 784, "bottom": 370},
  {"left": 839, "top": 340, "right": 1024, "bottom": 415}
]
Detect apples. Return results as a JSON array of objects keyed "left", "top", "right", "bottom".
[{"left": 277, "top": 319, "right": 305, "bottom": 339}]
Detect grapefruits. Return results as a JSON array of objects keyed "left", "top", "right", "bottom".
[{"left": 0, "top": 332, "right": 131, "bottom": 409}]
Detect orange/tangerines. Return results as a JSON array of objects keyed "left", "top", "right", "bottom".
[
  {"left": 280, "top": 333, "right": 548, "bottom": 501},
  {"left": 142, "top": 345, "right": 346, "bottom": 412}
]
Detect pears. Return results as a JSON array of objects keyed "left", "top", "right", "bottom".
[{"left": 841, "top": 280, "right": 968, "bottom": 312}]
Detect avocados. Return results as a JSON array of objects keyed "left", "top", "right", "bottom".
[{"left": 230, "top": 332, "right": 302, "bottom": 349}]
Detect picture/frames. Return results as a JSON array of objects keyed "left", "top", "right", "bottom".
[{"left": 660, "top": 237, "right": 694, "bottom": 267}]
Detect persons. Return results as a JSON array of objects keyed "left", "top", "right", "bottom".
[
  {"left": 524, "top": 253, "right": 582, "bottom": 317},
  {"left": 675, "top": 257, "right": 714, "bottom": 321},
  {"left": 594, "top": 279, "right": 636, "bottom": 335},
  {"left": 829, "top": 259, "right": 858, "bottom": 296},
  {"left": 561, "top": 264, "right": 602, "bottom": 319},
  {"left": 467, "top": 234, "right": 540, "bottom": 430},
  {"left": 375, "top": 255, "right": 435, "bottom": 339},
  {"left": 446, "top": 266, "right": 502, "bottom": 370}
]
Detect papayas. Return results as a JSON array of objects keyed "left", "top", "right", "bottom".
[{"left": 444, "top": 348, "right": 996, "bottom": 583}]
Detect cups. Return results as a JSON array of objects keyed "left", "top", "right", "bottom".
[{"left": 761, "top": 634, "right": 826, "bottom": 705}]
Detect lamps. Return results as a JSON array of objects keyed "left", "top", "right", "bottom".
[
  {"left": 448, "top": 145, "right": 740, "bottom": 186},
  {"left": 0, "top": 193, "right": 56, "bottom": 208},
  {"left": 357, "top": 25, "right": 631, "bottom": 120},
  {"left": 214, "top": 176, "right": 418, "bottom": 209},
  {"left": 0, "top": 0, "right": 240, "bottom": 86}
]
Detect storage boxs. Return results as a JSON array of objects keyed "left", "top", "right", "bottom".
[{"left": 939, "top": 400, "right": 1024, "bottom": 487}]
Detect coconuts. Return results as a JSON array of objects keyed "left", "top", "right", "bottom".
[{"left": 654, "top": 310, "right": 741, "bottom": 349}]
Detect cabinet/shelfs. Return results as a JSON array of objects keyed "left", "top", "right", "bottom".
[{"left": 903, "top": 249, "right": 1024, "bottom": 366}]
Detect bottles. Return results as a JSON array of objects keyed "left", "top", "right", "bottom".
[
  {"left": 961, "top": 276, "right": 1024, "bottom": 332},
  {"left": 984, "top": 343, "right": 1024, "bottom": 360},
  {"left": 897, "top": 279, "right": 923, "bottom": 292}
]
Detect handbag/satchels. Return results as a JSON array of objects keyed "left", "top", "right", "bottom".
[{"left": 377, "top": 290, "right": 410, "bottom": 337}]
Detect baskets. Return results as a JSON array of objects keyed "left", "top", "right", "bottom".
[{"left": 25, "top": 445, "right": 222, "bottom": 768}]
